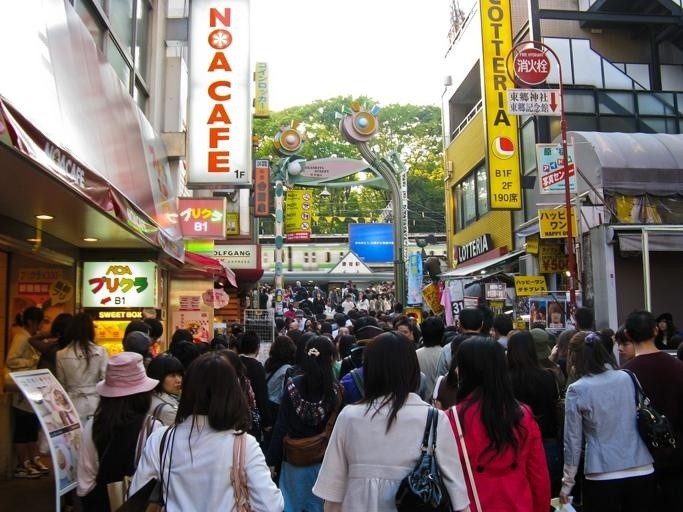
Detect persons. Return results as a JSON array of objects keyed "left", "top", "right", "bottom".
[
  {"left": 506, "top": 331, "right": 559, "bottom": 427},
  {"left": 123, "top": 320, "right": 153, "bottom": 344},
  {"left": 548, "top": 302, "right": 564, "bottom": 329},
  {"left": 560, "top": 331, "right": 655, "bottom": 512},
  {"left": 124, "top": 353, "right": 285, "bottom": 511},
  {"left": 77, "top": 352, "right": 163, "bottom": 511},
  {"left": 168, "top": 323, "right": 243, "bottom": 367},
  {"left": 415, "top": 316, "right": 445, "bottom": 393},
  {"left": 653, "top": 313, "right": 682, "bottom": 349},
  {"left": 442, "top": 338, "right": 551, "bottom": 511},
  {"left": 600, "top": 328, "right": 619, "bottom": 363},
  {"left": 548, "top": 329, "right": 579, "bottom": 376},
  {"left": 433, "top": 309, "right": 513, "bottom": 378},
  {"left": 265, "top": 335, "right": 297, "bottom": 423},
  {"left": 122, "top": 331, "right": 153, "bottom": 370},
  {"left": 614, "top": 324, "right": 636, "bottom": 364},
  {"left": 26, "top": 313, "right": 71, "bottom": 455},
  {"left": 248, "top": 280, "right": 424, "bottom": 358},
  {"left": 531, "top": 302, "right": 546, "bottom": 322},
  {"left": 311, "top": 330, "right": 471, "bottom": 512},
  {"left": 56, "top": 311, "right": 109, "bottom": 428},
  {"left": 529, "top": 328, "right": 565, "bottom": 387},
  {"left": 237, "top": 331, "right": 274, "bottom": 430},
  {"left": 620, "top": 310, "right": 683, "bottom": 511},
  {"left": 338, "top": 361, "right": 428, "bottom": 406},
  {"left": 146, "top": 353, "right": 185, "bottom": 426},
  {"left": 264, "top": 337, "right": 345, "bottom": 511},
  {"left": 5, "top": 306, "right": 51, "bottom": 479},
  {"left": 219, "top": 350, "right": 263, "bottom": 443},
  {"left": 144, "top": 319, "right": 163, "bottom": 342}
]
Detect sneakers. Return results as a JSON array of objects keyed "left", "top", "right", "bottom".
[
  {"left": 33, "top": 456, "right": 49, "bottom": 475},
  {"left": 14, "top": 459, "right": 39, "bottom": 477}
]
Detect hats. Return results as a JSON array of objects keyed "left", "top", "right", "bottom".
[
  {"left": 295, "top": 309, "right": 304, "bottom": 317},
  {"left": 530, "top": 328, "right": 551, "bottom": 359},
  {"left": 94, "top": 351, "right": 159, "bottom": 398}
]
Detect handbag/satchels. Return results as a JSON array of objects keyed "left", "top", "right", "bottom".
[
  {"left": 395, "top": 406, "right": 454, "bottom": 512},
  {"left": 621, "top": 367, "right": 677, "bottom": 458},
  {"left": 116, "top": 423, "right": 176, "bottom": 511},
  {"left": 282, "top": 391, "right": 342, "bottom": 466},
  {"left": 232, "top": 429, "right": 250, "bottom": 512},
  {"left": 108, "top": 413, "right": 154, "bottom": 508}
]
